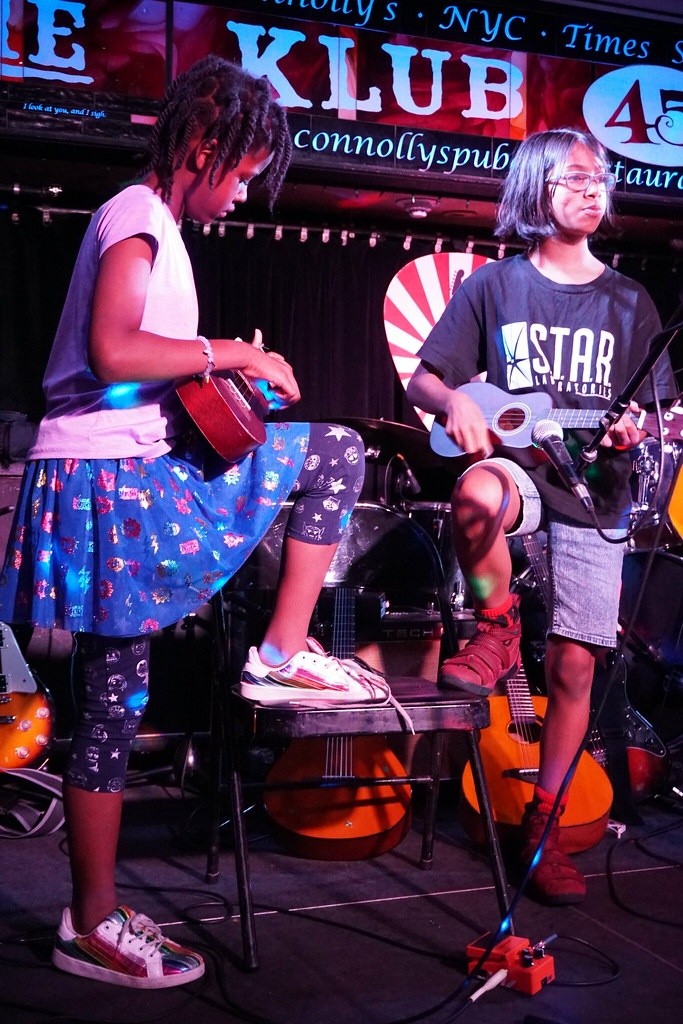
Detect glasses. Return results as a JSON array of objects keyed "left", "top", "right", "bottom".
[{"left": 545, "top": 172, "right": 616, "bottom": 192}]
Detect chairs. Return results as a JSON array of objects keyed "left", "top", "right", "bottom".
[{"left": 181, "top": 500, "right": 516, "bottom": 967}]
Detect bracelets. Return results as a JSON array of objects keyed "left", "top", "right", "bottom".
[{"left": 196, "top": 336, "right": 214, "bottom": 377}]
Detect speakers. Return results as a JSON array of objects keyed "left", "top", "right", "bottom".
[
  {"left": 360, "top": 623, "right": 479, "bottom": 782},
  {"left": 0, "top": 463, "right": 26, "bottom": 576}
]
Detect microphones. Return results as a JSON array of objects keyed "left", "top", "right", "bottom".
[
  {"left": 663, "top": 670, "right": 683, "bottom": 690},
  {"left": 395, "top": 453, "right": 422, "bottom": 494},
  {"left": 530, "top": 420, "right": 594, "bottom": 514}
]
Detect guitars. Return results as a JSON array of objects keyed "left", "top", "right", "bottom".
[
  {"left": 521, "top": 532, "right": 674, "bottom": 809},
  {"left": 258, "top": 580, "right": 415, "bottom": 861},
  {"left": 454, "top": 649, "right": 615, "bottom": 860},
  {"left": 429, "top": 379, "right": 683, "bottom": 470},
  {"left": 0, "top": 619, "right": 58, "bottom": 772},
  {"left": 174, "top": 342, "right": 277, "bottom": 466}
]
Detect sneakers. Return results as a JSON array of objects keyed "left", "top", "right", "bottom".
[
  {"left": 240, "top": 636, "right": 392, "bottom": 710},
  {"left": 440, "top": 624, "right": 521, "bottom": 695},
  {"left": 52, "top": 904, "right": 205, "bottom": 989},
  {"left": 522, "top": 812, "right": 586, "bottom": 903}
]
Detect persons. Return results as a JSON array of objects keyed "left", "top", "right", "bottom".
[
  {"left": 407, "top": 129, "right": 678, "bottom": 902},
  {"left": 0, "top": 55, "right": 391, "bottom": 989}
]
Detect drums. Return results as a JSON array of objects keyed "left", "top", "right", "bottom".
[
  {"left": 625, "top": 434, "right": 683, "bottom": 549},
  {"left": 401, "top": 498, "right": 471, "bottom": 616},
  {"left": 234, "top": 500, "right": 447, "bottom": 617}
]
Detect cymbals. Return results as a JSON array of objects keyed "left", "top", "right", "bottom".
[{"left": 308, "top": 415, "right": 431, "bottom": 466}]
